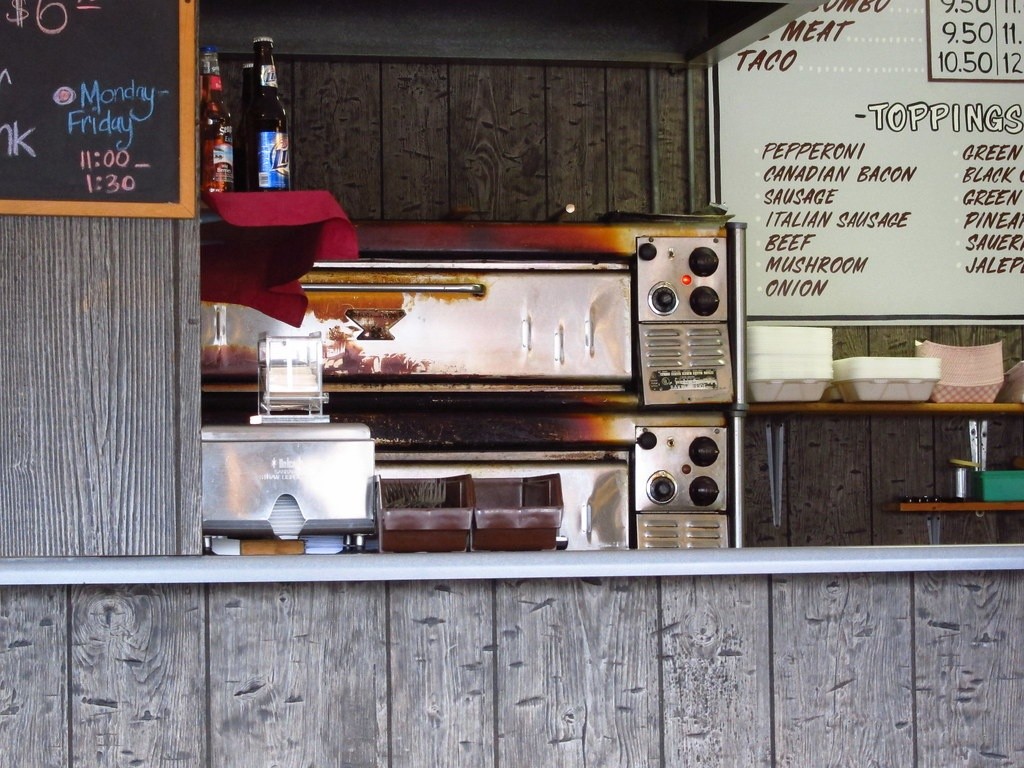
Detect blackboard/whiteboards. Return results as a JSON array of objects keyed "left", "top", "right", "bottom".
[{"left": 0, "top": 0, "right": 197, "bottom": 219}]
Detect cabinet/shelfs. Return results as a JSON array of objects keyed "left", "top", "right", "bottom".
[{"left": 743, "top": 400, "right": 1023, "bottom": 515}]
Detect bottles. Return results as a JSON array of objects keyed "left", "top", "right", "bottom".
[{"left": 193, "top": 25, "right": 293, "bottom": 193}]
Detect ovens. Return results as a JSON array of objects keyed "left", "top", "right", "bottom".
[{"left": 203, "top": 209, "right": 735, "bottom": 545}]
